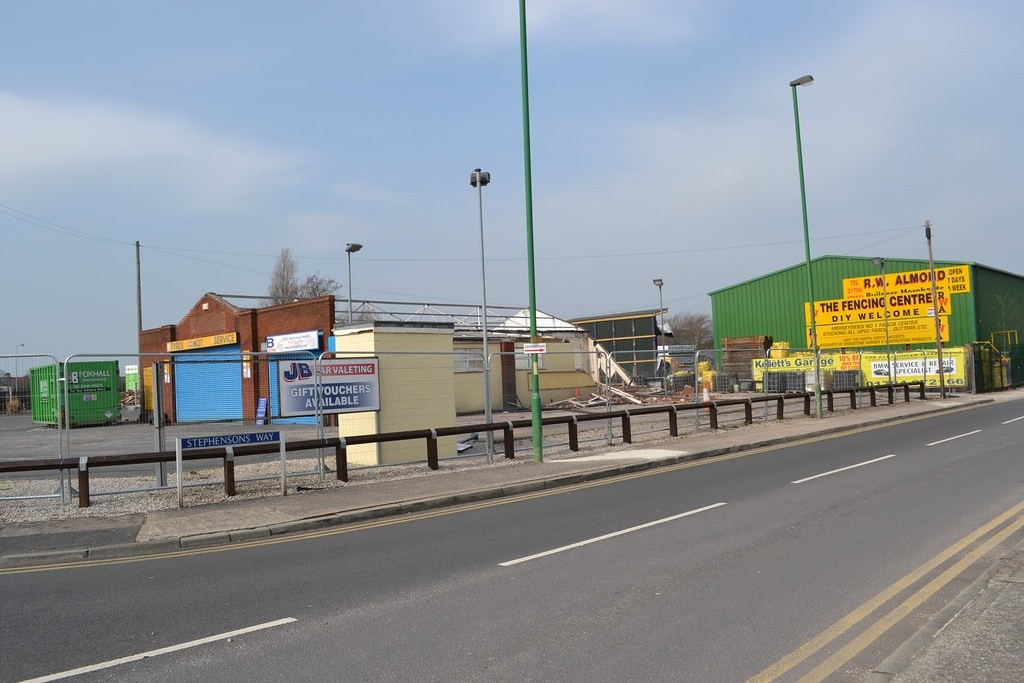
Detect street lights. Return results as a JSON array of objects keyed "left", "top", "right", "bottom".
[
  {"left": 15, "top": 343, "right": 24, "bottom": 397},
  {"left": 872, "top": 257, "right": 892, "bottom": 382},
  {"left": 469, "top": 168, "right": 492, "bottom": 452},
  {"left": 791, "top": 74, "right": 827, "bottom": 419},
  {"left": 653, "top": 278, "right": 669, "bottom": 399},
  {"left": 344, "top": 242, "right": 363, "bottom": 324}
]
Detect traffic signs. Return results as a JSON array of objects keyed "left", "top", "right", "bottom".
[{"left": 522, "top": 343, "right": 547, "bottom": 354}]
